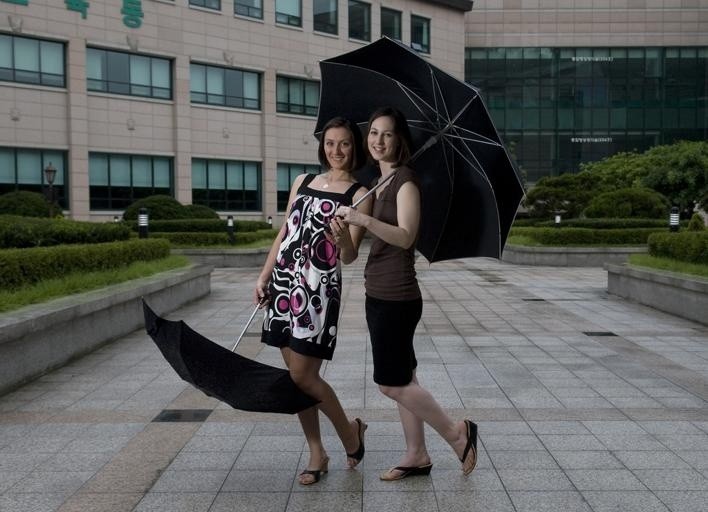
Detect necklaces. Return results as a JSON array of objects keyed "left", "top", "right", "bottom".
[{"left": 322, "top": 182, "right": 329, "bottom": 189}]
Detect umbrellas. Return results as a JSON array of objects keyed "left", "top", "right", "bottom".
[
  {"left": 141, "top": 292, "right": 322, "bottom": 414},
  {"left": 314, "top": 35, "right": 525, "bottom": 266}
]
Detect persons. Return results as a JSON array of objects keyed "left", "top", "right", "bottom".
[
  {"left": 254, "top": 117, "right": 367, "bottom": 485},
  {"left": 340, "top": 108, "right": 477, "bottom": 481}
]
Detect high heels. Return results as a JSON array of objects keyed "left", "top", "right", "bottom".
[
  {"left": 299, "top": 455, "right": 329, "bottom": 484},
  {"left": 346, "top": 418, "right": 368, "bottom": 469}
]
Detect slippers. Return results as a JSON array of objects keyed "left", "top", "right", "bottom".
[
  {"left": 379, "top": 462, "right": 433, "bottom": 481},
  {"left": 459, "top": 420, "right": 478, "bottom": 479}
]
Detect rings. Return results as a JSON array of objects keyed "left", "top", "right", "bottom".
[{"left": 335, "top": 237, "right": 342, "bottom": 241}]
